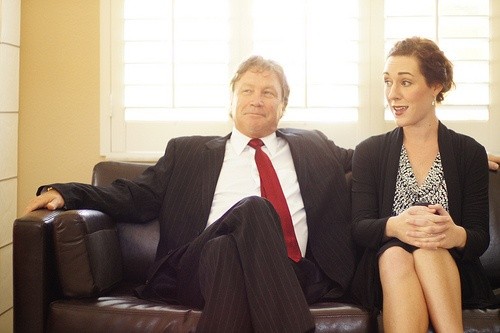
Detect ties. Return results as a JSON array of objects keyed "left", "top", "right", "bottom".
[{"left": 247, "top": 138, "right": 302, "bottom": 263}]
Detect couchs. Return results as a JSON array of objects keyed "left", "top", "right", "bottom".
[{"left": 12, "top": 161, "right": 500, "bottom": 333}]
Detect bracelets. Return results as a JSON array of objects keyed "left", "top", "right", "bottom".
[{"left": 47, "top": 186, "right": 54, "bottom": 191}]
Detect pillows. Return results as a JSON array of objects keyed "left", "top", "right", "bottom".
[{"left": 54, "top": 208, "right": 117, "bottom": 298}]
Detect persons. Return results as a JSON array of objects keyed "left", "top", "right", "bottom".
[
  {"left": 23, "top": 56, "right": 500, "bottom": 333},
  {"left": 351, "top": 36, "right": 491, "bottom": 333}
]
